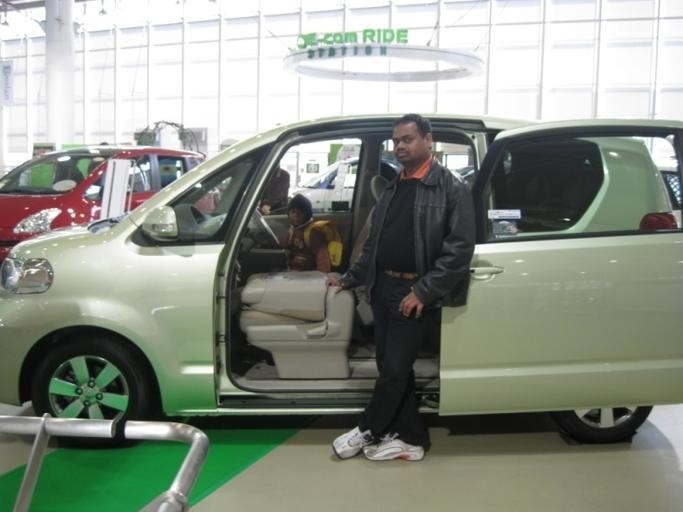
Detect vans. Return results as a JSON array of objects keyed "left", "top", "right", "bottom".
[{"left": 0, "top": 115, "right": 682, "bottom": 449}]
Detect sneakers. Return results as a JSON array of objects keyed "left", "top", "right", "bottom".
[
  {"left": 331, "top": 424, "right": 377, "bottom": 460},
  {"left": 363, "top": 432, "right": 425, "bottom": 462}
]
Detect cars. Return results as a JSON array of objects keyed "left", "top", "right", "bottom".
[
  {"left": 287, "top": 155, "right": 401, "bottom": 213},
  {"left": 0, "top": 146, "right": 222, "bottom": 264}
]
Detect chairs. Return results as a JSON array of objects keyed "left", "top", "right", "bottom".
[{"left": 348, "top": 173, "right": 390, "bottom": 269}]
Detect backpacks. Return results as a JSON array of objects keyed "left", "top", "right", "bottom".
[{"left": 287, "top": 217, "right": 344, "bottom": 267}]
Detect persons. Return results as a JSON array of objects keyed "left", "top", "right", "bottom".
[
  {"left": 258, "top": 160, "right": 290, "bottom": 214},
  {"left": 276, "top": 194, "right": 331, "bottom": 273},
  {"left": 321, "top": 115, "right": 476, "bottom": 460}
]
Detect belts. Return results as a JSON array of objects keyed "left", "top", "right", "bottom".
[{"left": 377, "top": 266, "right": 419, "bottom": 281}]
